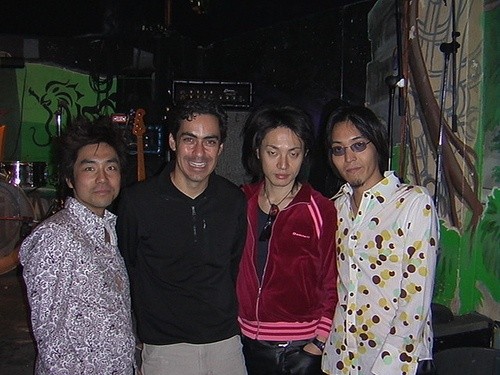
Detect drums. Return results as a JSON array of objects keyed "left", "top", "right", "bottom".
[
  {"left": 0, "top": 174, "right": 38, "bottom": 277},
  {"left": 3, "top": 160, "right": 48, "bottom": 190}
]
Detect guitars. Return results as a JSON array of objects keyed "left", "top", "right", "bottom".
[{"left": 130, "top": 108, "right": 147, "bottom": 180}]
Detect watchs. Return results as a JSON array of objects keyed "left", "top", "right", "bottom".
[{"left": 312, "top": 339, "right": 325, "bottom": 353}]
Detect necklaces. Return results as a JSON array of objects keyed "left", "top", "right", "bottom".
[
  {"left": 353, "top": 191, "right": 360, "bottom": 209},
  {"left": 259, "top": 178, "right": 296, "bottom": 206}
]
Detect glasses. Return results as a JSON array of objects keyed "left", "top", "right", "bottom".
[
  {"left": 259, "top": 204, "right": 280, "bottom": 242},
  {"left": 330, "top": 141, "right": 371, "bottom": 155}
]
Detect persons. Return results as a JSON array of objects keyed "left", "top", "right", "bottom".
[
  {"left": 322, "top": 105, "right": 441, "bottom": 375},
  {"left": 110, "top": 98, "right": 248, "bottom": 375},
  {"left": 237, "top": 106, "right": 338, "bottom": 375},
  {"left": 18, "top": 113, "right": 136, "bottom": 375}
]
